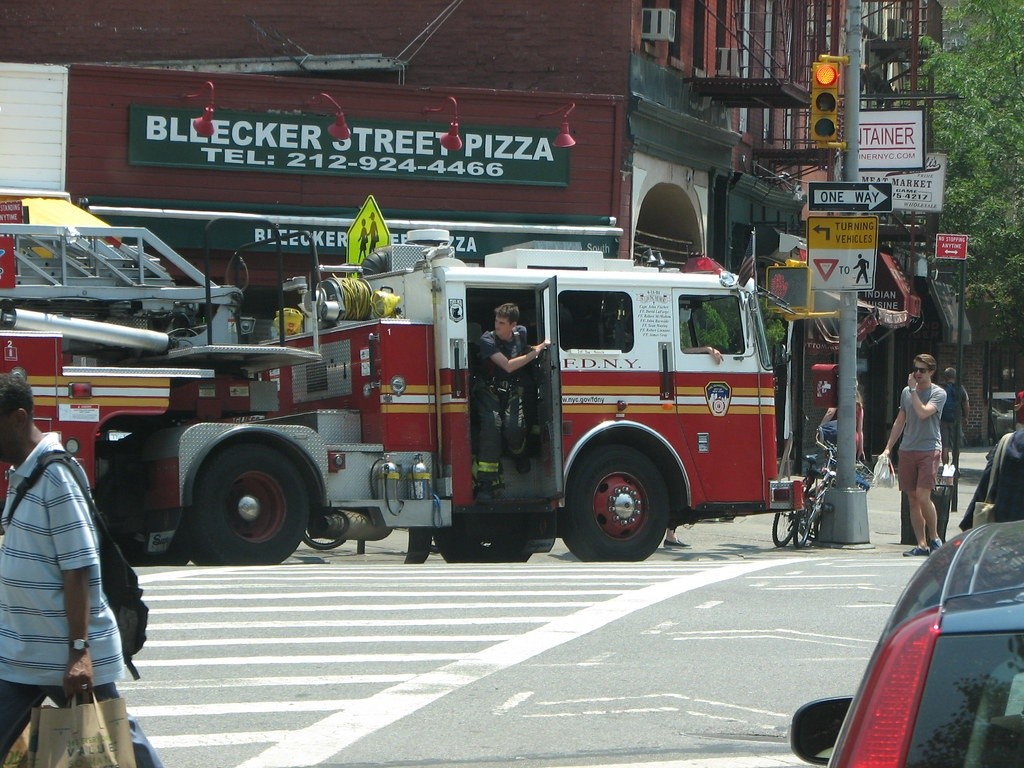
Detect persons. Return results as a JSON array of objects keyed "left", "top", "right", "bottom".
[
  {"left": 877, "top": 353, "right": 947, "bottom": 558},
  {"left": 816, "top": 370, "right": 865, "bottom": 477},
  {"left": 474, "top": 303, "right": 551, "bottom": 497},
  {"left": 681, "top": 346, "right": 724, "bottom": 364},
  {"left": 941, "top": 367, "right": 969, "bottom": 473},
  {"left": 958, "top": 429, "right": 1023, "bottom": 529},
  {"left": 1014, "top": 391, "right": 1024, "bottom": 431},
  {"left": 0, "top": 374, "right": 157, "bottom": 768},
  {"left": 664, "top": 526, "right": 690, "bottom": 549}
]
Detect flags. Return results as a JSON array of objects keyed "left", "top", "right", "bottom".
[{"left": 738, "top": 233, "right": 754, "bottom": 287}]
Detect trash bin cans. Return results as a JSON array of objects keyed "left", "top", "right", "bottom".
[{"left": 901, "top": 485, "right": 952, "bottom": 544}]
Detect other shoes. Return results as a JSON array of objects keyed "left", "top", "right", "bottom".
[
  {"left": 475, "top": 487, "right": 493, "bottom": 504},
  {"left": 515, "top": 454, "right": 530, "bottom": 472},
  {"left": 664, "top": 539, "right": 691, "bottom": 548}
]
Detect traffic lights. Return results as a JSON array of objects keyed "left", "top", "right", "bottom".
[
  {"left": 765, "top": 264, "right": 812, "bottom": 313},
  {"left": 811, "top": 62, "right": 839, "bottom": 141}
]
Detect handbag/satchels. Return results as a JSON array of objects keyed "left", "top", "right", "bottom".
[
  {"left": 872, "top": 452, "right": 895, "bottom": 488},
  {"left": 101, "top": 534, "right": 148, "bottom": 680},
  {"left": 973, "top": 502, "right": 997, "bottom": 528},
  {"left": 27, "top": 691, "right": 137, "bottom": 768},
  {"left": 818, "top": 420, "right": 837, "bottom": 445},
  {"left": 0, "top": 721, "right": 31, "bottom": 768}
]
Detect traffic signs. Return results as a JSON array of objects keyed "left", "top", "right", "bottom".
[
  {"left": 807, "top": 182, "right": 893, "bottom": 212},
  {"left": 935, "top": 234, "right": 968, "bottom": 260},
  {"left": 806, "top": 216, "right": 879, "bottom": 292}
]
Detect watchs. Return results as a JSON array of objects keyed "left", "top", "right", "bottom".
[
  {"left": 68, "top": 638, "right": 90, "bottom": 652},
  {"left": 908, "top": 387, "right": 916, "bottom": 393}
]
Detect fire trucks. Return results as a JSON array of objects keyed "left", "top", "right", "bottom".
[{"left": 0, "top": 217, "right": 805, "bottom": 568}]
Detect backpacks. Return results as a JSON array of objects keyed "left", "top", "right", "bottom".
[{"left": 938, "top": 381, "right": 963, "bottom": 423}]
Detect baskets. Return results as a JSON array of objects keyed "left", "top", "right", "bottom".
[{"left": 857, "top": 460, "right": 875, "bottom": 492}]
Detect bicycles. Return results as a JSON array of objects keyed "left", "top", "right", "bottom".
[{"left": 773, "top": 433, "right": 874, "bottom": 548}]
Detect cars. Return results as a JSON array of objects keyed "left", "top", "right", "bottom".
[
  {"left": 991, "top": 405, "right": 1015, "bottom": 435},
  {"left": 789, "top": 520, "right": 1024, "bottom": 768}
]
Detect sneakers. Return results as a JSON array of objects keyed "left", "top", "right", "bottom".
[
  {"left": 930, "top": 537, "right": 942, "bottom": 555},
  {"left": 903, "top": 544, "right": 930, "bottom": 556}
]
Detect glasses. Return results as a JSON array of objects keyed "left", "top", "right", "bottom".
[{"left": 913, "top": 367, "right": 930, "bottom": 373}]
